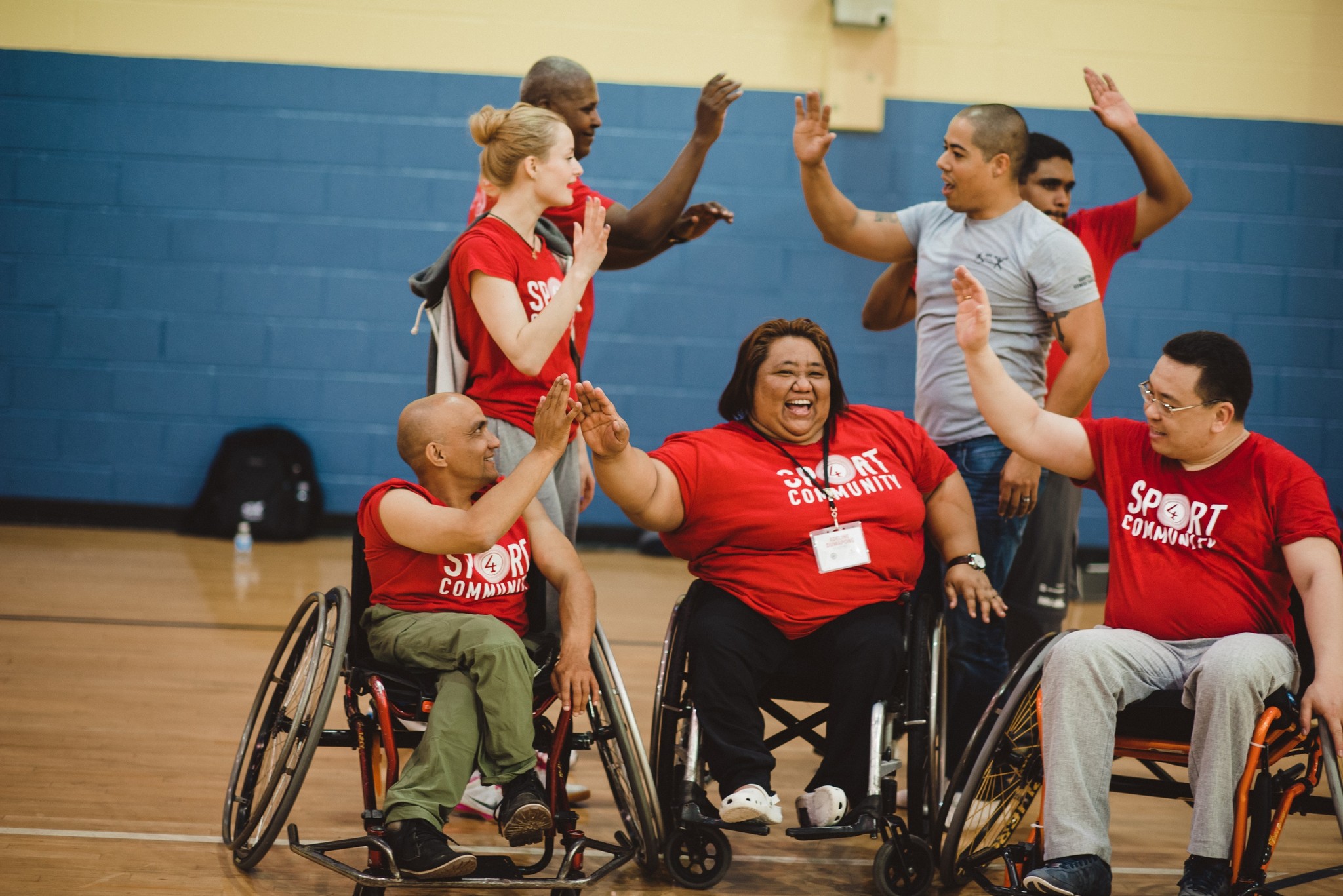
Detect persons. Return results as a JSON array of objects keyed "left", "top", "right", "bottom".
[
  {"left": 948, "top": 265, "right": 1343, "bottom": 896},
  {"left": 406, "top": 57, "right": 1192, "bottom": 831},
  {"left": 354, "top": 375, "right": 597, "bottom": 882}
]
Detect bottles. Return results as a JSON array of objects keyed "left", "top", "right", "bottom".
[{"left": 233, "top": 521, "right": 253, "bottom": 586}]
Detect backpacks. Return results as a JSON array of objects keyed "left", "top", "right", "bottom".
[{"left": 183, "top": 424, "right": 324, "bottom": 542}]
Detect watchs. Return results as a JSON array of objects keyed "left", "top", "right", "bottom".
[
  {"left": 664, "top": 230, "right": 687, "bottom": 248},
  {"left": 947, "top": 553, "right": 986, "bottom": 571}
]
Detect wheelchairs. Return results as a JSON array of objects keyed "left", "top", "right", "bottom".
[
  {"left": 649, "top": 527, "right": 941, "bottom": 896},
  {"left": 221, "top": 514, "right": 665, "bottom": 896},
  {"left": 940, "top": 609, "right": 1343, "bottom": 896}
]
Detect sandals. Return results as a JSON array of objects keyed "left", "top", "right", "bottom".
[
  {"left": 720, "top": 784, "right": 783, "bottom": 824},
  {"left": 795, "top": 785, "right": 848, "bottom": 827}
]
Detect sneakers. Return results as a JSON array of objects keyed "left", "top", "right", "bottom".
[
  {"left": 1023, "top": 854, "right": 1113, "bottom": 896},
  {"left": 386, "top": 816, "right": 477, "bottom": 881},
  {"left": 1176, "top": 855, "right": 1233, "bottom": 896},
  {"left": 493, "top": 769, "right": 553, "bottom": 848}
]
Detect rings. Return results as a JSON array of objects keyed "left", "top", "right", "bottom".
[
  {"left": 965, "top": 295, "right": 971, "bottom": 299},
  {"left": 1022, "top": 496, "right": 1030, "bottom": 502}
]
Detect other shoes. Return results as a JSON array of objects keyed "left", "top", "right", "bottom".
[
  {"left": 924, "top": 790, "right": 1010, "bottom": 831},
  {"left": 534, "top": 745, "right": 577, "bottom": 767},
  {"left": 455, "top": 770, "right": 501, "bottom": 823},
  {"left": 897, "top": 777, "right": 952, "bottom": 809},
  {"left": 536, "top": 767, "right": 591, "bottom": 803}
]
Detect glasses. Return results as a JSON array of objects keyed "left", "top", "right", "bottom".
[{"left": 1138, "top": 380, "right": 1223, "bottom": 418}]
